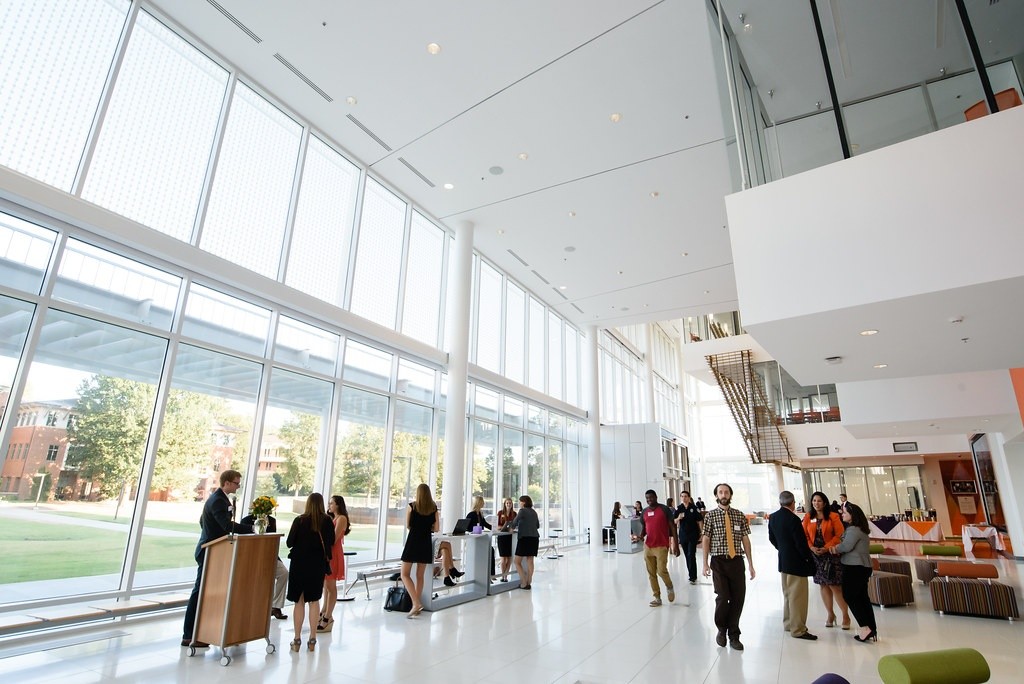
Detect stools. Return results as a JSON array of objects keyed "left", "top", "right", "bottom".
[
  {"left": 611, "top": 528, "right": 617, "bottom": 550},
  {"left": 602, "top": 526, "right": 616, "bottom": 552}
]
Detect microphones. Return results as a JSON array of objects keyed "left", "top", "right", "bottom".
[{"left": 232, "top": 496, "right": 237, "bottom": 511}]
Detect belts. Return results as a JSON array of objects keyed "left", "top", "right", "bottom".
[{"left": 715, "top": 554, "right": 742, "bottom": 559}]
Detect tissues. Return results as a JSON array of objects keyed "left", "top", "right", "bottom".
[{"left": 473, "top": 523, "right": 482, "bottom": 534}]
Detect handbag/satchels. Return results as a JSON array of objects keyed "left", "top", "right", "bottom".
[{"left": 384, "top": 576, "right": 413, "bottom": 612}]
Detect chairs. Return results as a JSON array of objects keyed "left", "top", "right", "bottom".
[
  {"left": 776, "top": 406, "right": 839, "bottom": 425},
  {"left": 689, "top": 331, "right": 702, "bottom": 342}
]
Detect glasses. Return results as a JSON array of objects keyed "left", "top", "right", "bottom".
[{"left": 227, "top": 479, "right": 241, "bottom": 487}]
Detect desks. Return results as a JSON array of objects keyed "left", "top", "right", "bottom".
[
  {"left": 483, "top": 531, "right": 527, "bottom": 595},
  {"left": 866, "top": 520, "right": 941, "bottom": 543},
  {"left": 616, "top": 517, "right": 644, "bottom": 554},
  {"left": 788, "top": 410, "right": 830, "bottom": 423},
  {"left": 409, "top": 531, "right": 490, "bottom": 612},
  {"left": 961, "top": 524, "right": 1002, "bottom": 557}
]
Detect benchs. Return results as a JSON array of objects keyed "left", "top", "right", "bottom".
[
  {"left": 496, "top": 543, "right": 563, "bottom": 565},
  {"left": 863, "top": 543, "right": 912, "bottom": 579},
  {"left": 929, "top": 561, "right": 1019, "bottom": 621},
  {"left": 866, "top": 568, "right": 915, "bottom": 608},
  {"left": 346, "top": 556, "right": 462, "bottom": 601},
  {"left": 914, "top": 544, "right": 973, "bottom": 585}
]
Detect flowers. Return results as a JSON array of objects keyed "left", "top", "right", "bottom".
[{"left": 251, "top": 497, "right": 276, "bottom": 513}]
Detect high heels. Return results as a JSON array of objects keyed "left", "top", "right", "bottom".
[
  {"left": 854, "top": 629, "right": 877, "bottom": 642},
  {"left": 841, "top": 616, "right": 850, "bottom": 630},
  {"left": 449, "top": 568, "right": 465, "bottom": 579},
  {"left": 316, "top": 612, "right": 335, "bottom": 633},
  {"left": 825, "top": 613, "right": 837, "bottom": 627},
  {"left": 307, "top": 637, "right": 317, "bottom": 651},
  {"left": 443, "top": 576, "right": 456, "bottom": 587},
  {"left": 289, "top": 637, "right": 301, "bottom": 651}
]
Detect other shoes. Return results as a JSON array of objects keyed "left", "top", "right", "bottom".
[
  {"left": 649, "top": 598, "right": 662, "bottom": 607},
  {"left": 666, "top": 585, "right": 674, "bottom": 602},
  {"left": 271, "top": 606, "right": 288, "bottom": 619},
  {"left": 715, "top": 627, "right": 727, "bottom": 647},
  {"left": 181, "top": 637, "right": 210, "bottom": 647},
  {"left": 796, "top": 631, "right": 818, "bottom": 640},
  {"left": 489, "top": 573, "right": 534, "bottom": 589},
  {"left": 728, "top": 634, "right": 744, "bottom": 650},
  {"left": 407, "top": 604, "right": 425, "bottom": 618},
  {"left": 689, "top": 576, "right": 697, "bottom": 584}
]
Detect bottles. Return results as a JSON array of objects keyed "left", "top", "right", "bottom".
[
  {"left": 899, "top": 514, "right": 926, "bottom": 521},
  {"left": 932, "top": 516, "right": 935, "bottom": 522}
]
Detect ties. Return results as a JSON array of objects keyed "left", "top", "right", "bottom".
[
  {"left": 722, "top": 510, "right": 735, "bottom": 560},
  {"left": 842, "top": 503, "right": 844, "bottom": 514}
]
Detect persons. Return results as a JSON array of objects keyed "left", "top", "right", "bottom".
[
  {"left": 672, "top": 491, "right": 703, "bottom": 584},
  {"left": 610, "top": 496, "right": 706, "bottom": 554},
  {"left": 838, "top": 494, "right": 852, "bottom": 527},
  {"left": 800, "top": 492, "right": 850, "bottom": 630},
  {"left": 828, "top": 503, "right": 878, "bottom": 643},
  {"left": 498, "top": 498, "right": 517, "bottom": 582},
  {"left": 767, "top": 490, "right": 818, "bottom": 640},
  {"left": 239, "top": 496, "right": 288, "bottom": 619},
  {"left": 285, "top": 492, "right": 335, "bottom": 652},
  {"left": 465, "top": 496, "right": 497, "bottom": 583},
  {"left": 831, "top": 500, "right": 841, "bottom": 513},
  {"left": 399, "top": 484, "right": 439, "bottom": 618},
  {"left": 316, "top": 496, "right": 351, "bottom": 633},
  {"left": 700, "top": 483, "right": 756, "bottom": 651},
  {"left": 630, "top": 489, "right": 679, "bottom": 607},
  {"left": 508, "top": 495, "right": 540, "bottom": 589},
  {"left": 434, "top": 539, "right": 465, "bottom": 586},
  {"left": 179, "top": 469, "right": 257, "bottom": 647}
]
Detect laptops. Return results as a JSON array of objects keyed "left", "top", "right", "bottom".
[
  {"left": 500, "top": 521, "right": 513, "bottom": 532},
  {"left": 443, "top": 519, "right": 471, "bottom": 535}
]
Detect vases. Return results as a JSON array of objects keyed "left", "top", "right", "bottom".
[{"left": 252, "top": 512, "right": 268, "bottom": 535}]
